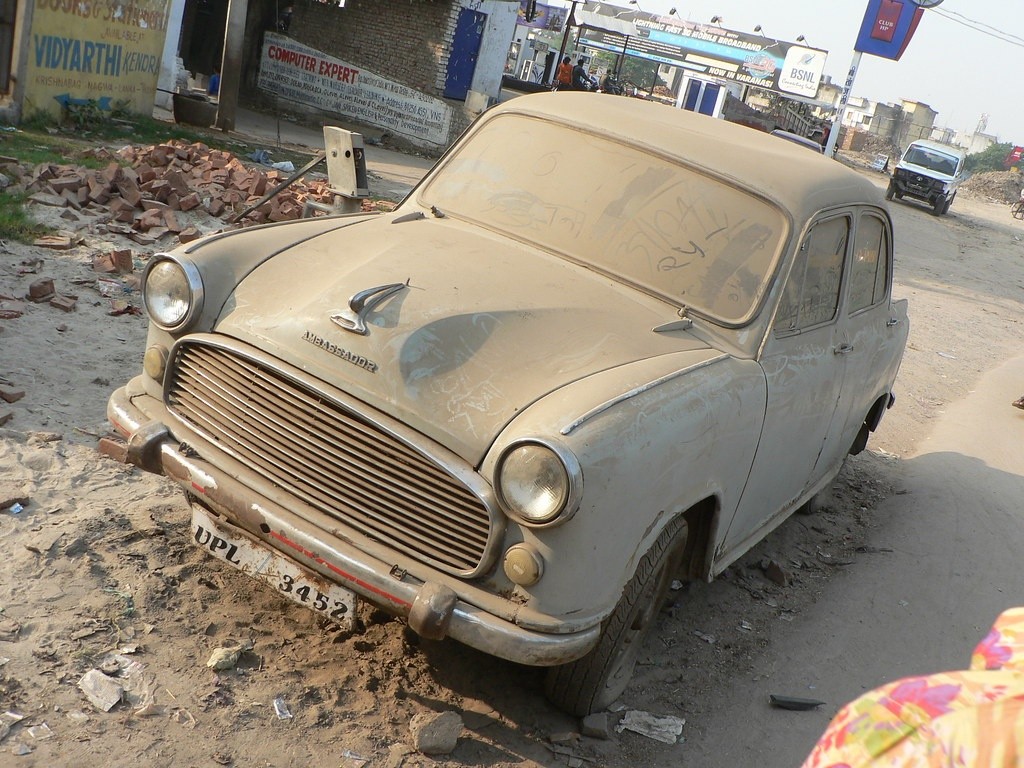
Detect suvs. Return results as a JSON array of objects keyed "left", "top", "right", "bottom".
[{"left": 885, "top": 139, "right": 967, "bottom": 217}]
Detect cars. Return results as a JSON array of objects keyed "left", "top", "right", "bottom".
[{"left": 97, "top": 91, "right": 910, "bottom": 712}]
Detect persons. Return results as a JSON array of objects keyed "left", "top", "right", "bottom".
[
  {"left": 556, "top": 57, "right": 593, "bottom": 90},
  {"left": 600, "top": 69, "right": 617, "bottom": 94},
  {"left": 803, "top": 607, "right": 1023, "bottom": 768},
  {"left": 207, "top": 62, "right": 221, "bottom": 97}
]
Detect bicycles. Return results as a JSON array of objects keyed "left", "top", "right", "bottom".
[{"left": 1010, "top": 198, "right": 1024, "bottom": 220}]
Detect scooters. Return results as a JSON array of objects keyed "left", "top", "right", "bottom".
[{"left": 551, "top": 80, "right": 624, "bottom": 96}]
